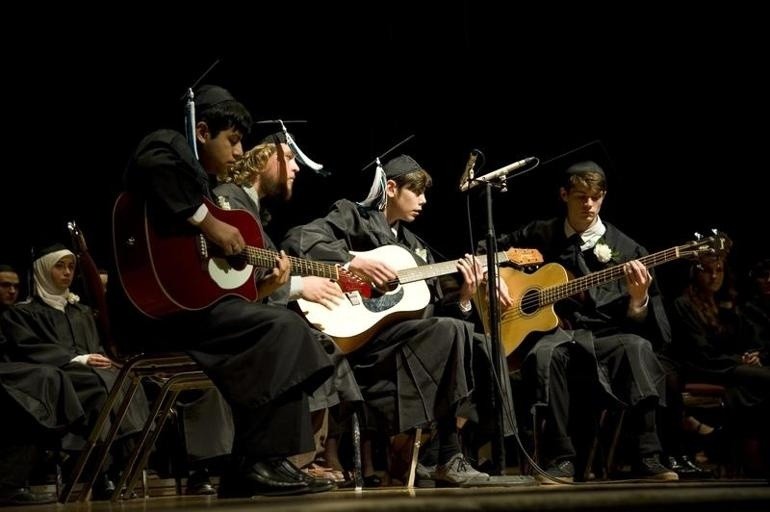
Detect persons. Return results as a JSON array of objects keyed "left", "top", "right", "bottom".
[
  {"left": 1, "top": 244, "right": 238, "bottom": 501},
  {"left": 126, "top": 59, "right": 335, "bottom": 498},
  {"left": 477, "top": 160, "right": 769, "bottom": 487},
  {"left": 283, "top": 133, "right": 493, "bottom": 489},
  {"left": 210, "top": 117, "right": 363, "bottom": 490}
]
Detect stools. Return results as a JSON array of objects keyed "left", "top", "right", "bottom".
[
  {"left": 108, "top": 369, "right": 214, "bottom": 504},
  {"left": 678, "top": 379, "right": 749, "bottom": 481}
]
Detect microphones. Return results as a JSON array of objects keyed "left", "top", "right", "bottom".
[{"left": 459, "top": 157, "right": 536, "bottom": 192}]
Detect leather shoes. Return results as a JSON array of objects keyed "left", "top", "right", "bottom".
[
  {"left": 1, "top": 466, "right": 139, "bottom": 505},
  {"left": 216, "top": 454, "right": 355, "bottom": 499},
  {"left": 184, "top": 470, "right": 215, "bottom": 496}
]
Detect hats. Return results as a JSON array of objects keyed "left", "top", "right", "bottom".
[
  {"left": 540, "top": 140, "right": 612, "bottom": 178},
  {"left": 249, "top": 114, "right": 313, "bottom": 150},
  {"left": 173, "top": 59, "right": 236, "bottom": 119},
  {"left": 359, "top": 130, "right": 423, "bottom": 181},
  {"left": 28, "top": 223, "right": 67, "bottom": 264}
]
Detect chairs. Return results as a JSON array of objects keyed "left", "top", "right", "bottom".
[{"left": 56, "top": 218, "right": 214, "bottom": 506}]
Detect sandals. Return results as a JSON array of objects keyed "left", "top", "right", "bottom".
[{"left": 361, "top": 469, "right": 383, "bottom": 487}]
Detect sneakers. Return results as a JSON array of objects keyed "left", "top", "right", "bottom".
[
  {"left": 534, "top": 460, "right": 576, "bottom": 485},
  {"left": 413, "top": 450, "right": 491, "bottom": 489},
  {"left": 631, "top": 423, "right": 720, "bottom": 482}
]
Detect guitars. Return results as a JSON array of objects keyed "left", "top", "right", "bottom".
[
  {"left": 480, "top": 228, "right": 734, "bottom": 358},
  {"left": 292, "top": 244, "right": 545, "bottom": 352},
  {"left": 112, "top": 182, "right": 372, "bottom": 317}
]
